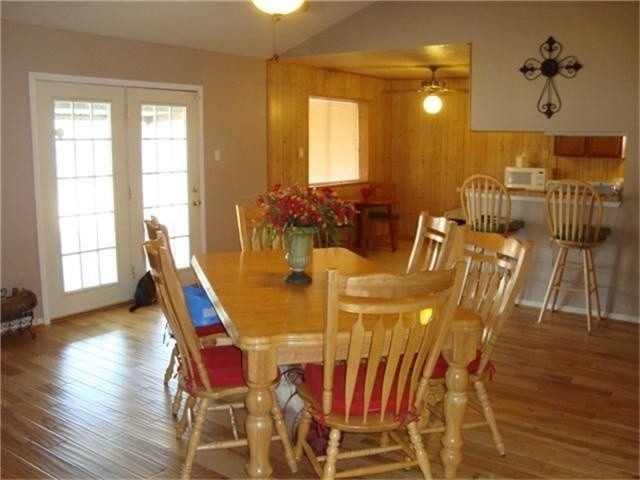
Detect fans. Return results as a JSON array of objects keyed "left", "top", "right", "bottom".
[{"left": 380, "top": 66, "right": 469, "bottom": 96}]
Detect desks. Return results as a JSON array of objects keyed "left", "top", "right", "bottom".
[{"left": 353, "top": 197, "right": 398, "bottom": 259}]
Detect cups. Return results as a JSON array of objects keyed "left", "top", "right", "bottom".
[{"left": 515, "top": 156, "right": 528, "bottom": 167}]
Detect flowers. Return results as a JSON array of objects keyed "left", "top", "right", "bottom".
[{"left": 247, "top": 179, "right": 357, "bottom": 256}]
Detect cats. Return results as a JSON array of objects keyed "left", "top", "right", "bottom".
[{"left": 130, "top": 271, "right": 157, "bottom": 312}]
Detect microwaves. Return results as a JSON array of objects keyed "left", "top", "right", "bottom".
[{"left": 503, "top": 166, "right": 553, "bottom": 192}]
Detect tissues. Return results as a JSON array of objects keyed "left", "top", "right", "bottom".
[{"left": 515, "top": 153, "right": 530, "bottom": 168}]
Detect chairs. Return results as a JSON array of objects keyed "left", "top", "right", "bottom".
[
  {"left": 454, "top": 172, "right": 526, "bottom": 311},
  {"left": 234, "top": 204, "right": 290, "bottom": 254},
  {"left": 142, "top": 216, "right": 298, "bottom": 480},
  {"left": 289, "top": 213, "right": 529, "bottom": 480},
  {"left": 536, "top": 178, "right": 614, "bottom": 335}
]
